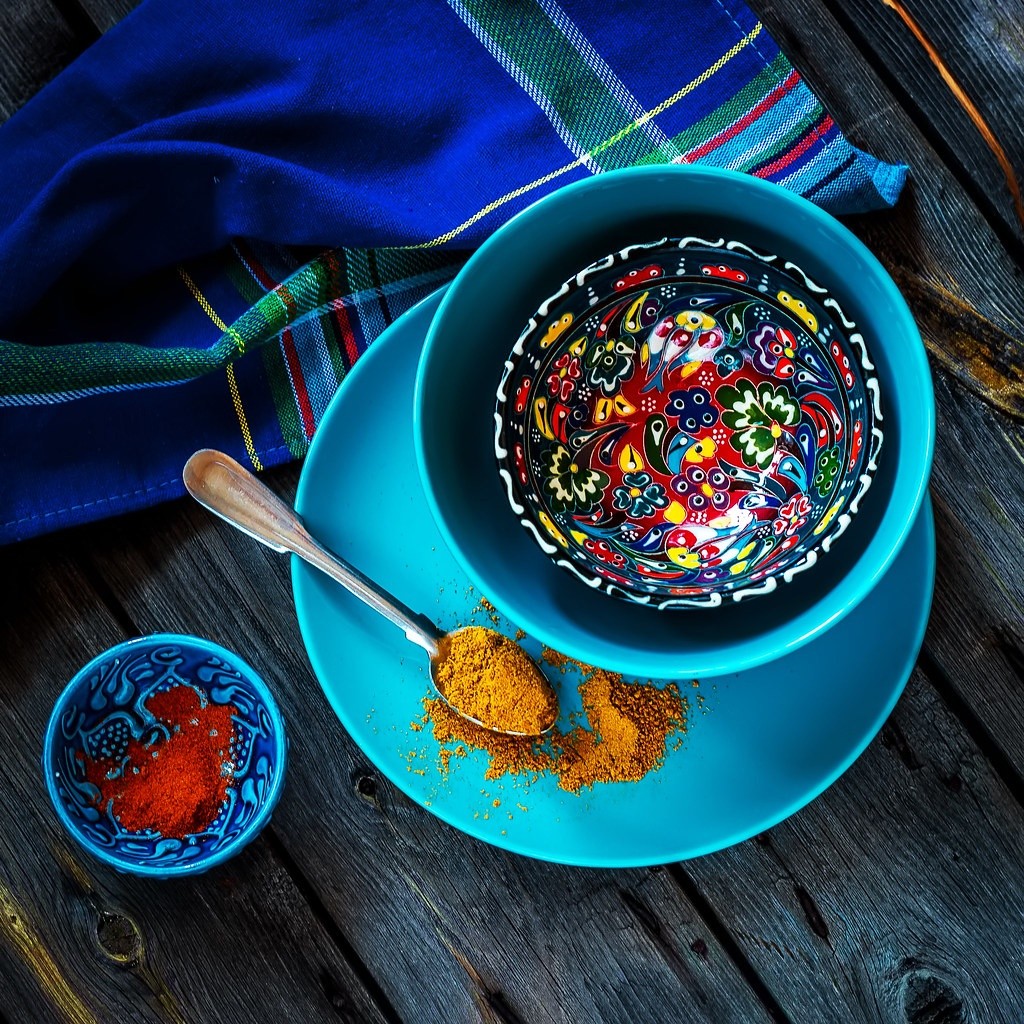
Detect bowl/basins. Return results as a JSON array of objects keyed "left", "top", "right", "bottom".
[
  {"left": 488, "top": 232, "right": 893, "bottom": 611},
  {"left": 410, "top": 160, "right": 939, "bottom": 685},
  {"left": 42, "top": 635, "right": 286, "bottom": 879}
]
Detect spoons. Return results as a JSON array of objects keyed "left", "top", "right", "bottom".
[{"left": 175, "top": 443, "right": 562, "bottom": 739}]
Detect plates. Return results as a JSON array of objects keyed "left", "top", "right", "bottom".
[{"left": 286, "top": 277, "right": 941, "bottom": 874}]
